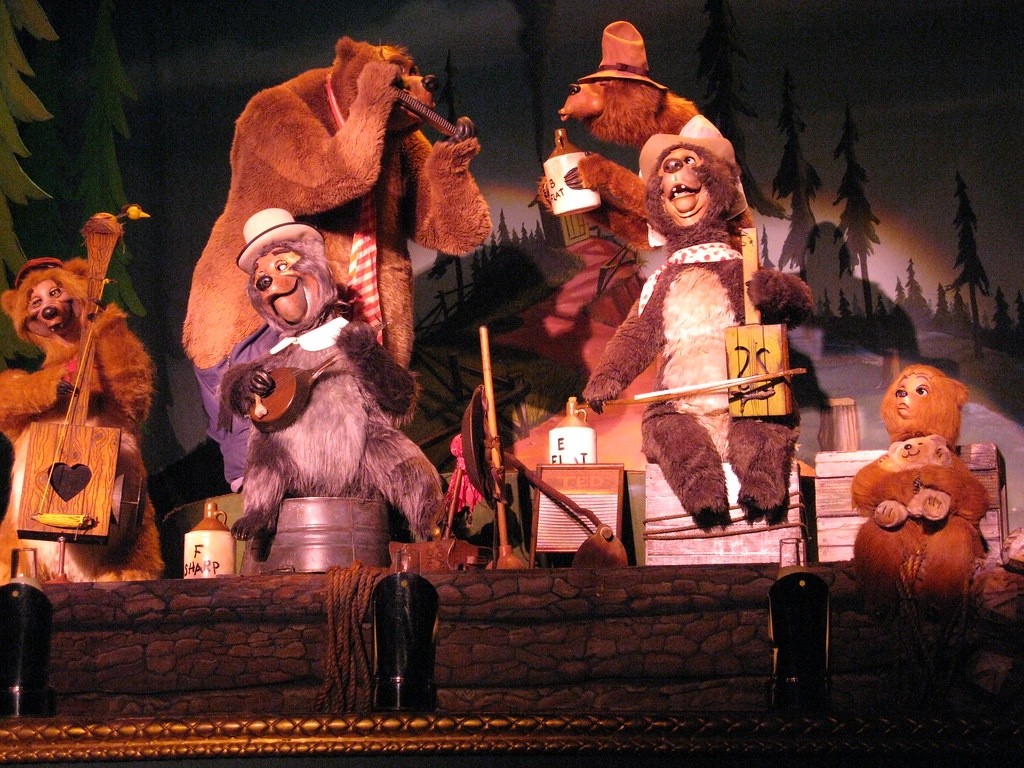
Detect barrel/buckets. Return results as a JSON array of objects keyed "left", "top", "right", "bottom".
[{"left": 239, "top": 496, "right": 391, "bottom": 575}]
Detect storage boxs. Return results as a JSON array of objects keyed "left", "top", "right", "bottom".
[
  {"left": 815, "top": 443, "right": 1010, "bottom": 563},
  {"left": 642, "top": 464, "right": 809, "bottom": 567}
]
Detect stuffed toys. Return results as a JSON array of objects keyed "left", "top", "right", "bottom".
[
  {"left": 873, "top": 435, "right": 953, "bottom": 528},
  {"left": 178, "top": 32, "right": 492, "bottom": 494},
  {"left": 216, "top": 208, "right": 444, "bottom": 545},
  {"left": 1, "top": 255, "right": 165, "bottom": 586},
  {"left": 580, "top": 114, "right": 814, "bottom": 528},
  {"left": 850, "top": 362, "right": 990, "bottom": 605},
  {"left": 536, "top": 20, "right": 754, "bottom": 376}
]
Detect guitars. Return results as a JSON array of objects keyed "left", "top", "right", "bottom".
[
  {"left": 14, "top": 210, "right": 125, "bottom": 545},
  {"left": 248, "top": 317, "right": 391, "bottom": 434}
]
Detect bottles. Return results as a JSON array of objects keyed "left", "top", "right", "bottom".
[
  {"left": 548, "top": 395, "right": 598, "bottom": 465},
  {"left": 184, "top": 502, "right": 237, "bottom": 578}
]
